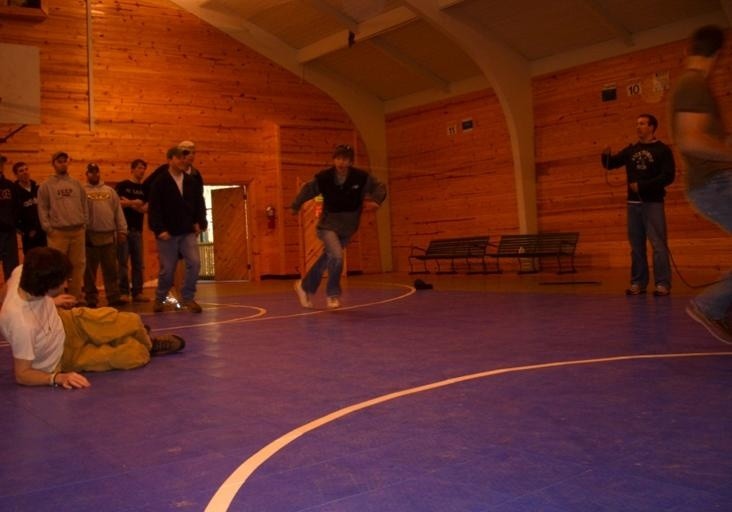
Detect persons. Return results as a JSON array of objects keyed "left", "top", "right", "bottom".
[
  {"left": 671, "top": 24, "right": 731, "bottom": 347},
  {"left": 600, "top": 113, "right": 675, "bottom": 296},
  {"left": 289, "top": 143, "right": 386, "bottom": 309},
  {"left": 2, "top": 245, "right": 185, "bottom": 390},
  {"left": 1, "top": 141, "right": 211, "bottom": 316}
]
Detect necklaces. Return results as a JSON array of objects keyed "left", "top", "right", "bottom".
[{"left": 28, "top": 295, "right": 52, "bottom": 338}]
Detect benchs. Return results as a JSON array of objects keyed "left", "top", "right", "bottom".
[
  {"left": 481, "top": 232, "right": 579, "bottom": 276},
  {"left": 407, "top": 236, "right": 490, "bottom": 275}
]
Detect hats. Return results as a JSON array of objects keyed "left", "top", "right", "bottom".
[
  {"left": 86, "top": 162, "right": 100, "bottom": 174},
  {"left": 167, "top": 141, "right": 196, "bottom": 159},
  {"left": 51, "top": 151, "right": 68, "bottom": 164},
  {"left": 331, "top": 142, "right": 355, "bottom": 159}
]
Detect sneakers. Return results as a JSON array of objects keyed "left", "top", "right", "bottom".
[
  {"left": 149, "top": 334, "right": 186, "bottom": 358},
  {"left": 685, "top": 297, "right": 732, "bottom": 346},
  {"left": 86, "top": 292, "right": 203, "bottom": 314},
  {"left": 653, "top": 285, "right": 671, "bottom": 297},
  {"left": 626, "top": 284, "right": 647, "bottom": 296},
  {"left": 325, "top": 296, "right": 341, "bottom": 309},
  {"left": 294, "top": 279, "right": 314, "bottom": 309}
]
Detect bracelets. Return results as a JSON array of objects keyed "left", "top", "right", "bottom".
[{"left": 48, "top": 371, "right": 62, "bottom": 385}]
